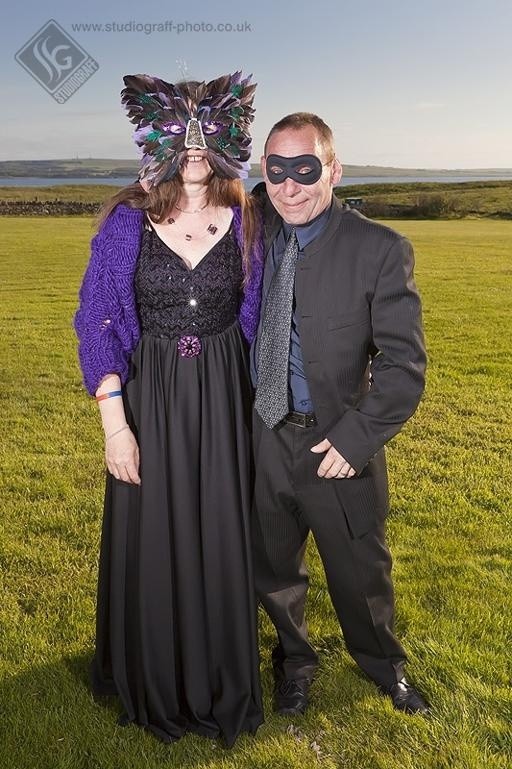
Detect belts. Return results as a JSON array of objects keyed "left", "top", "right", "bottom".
[{"left": 274, "top": 407, "right": 317, "bottom": 429}]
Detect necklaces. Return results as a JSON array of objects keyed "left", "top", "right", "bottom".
[
  {"left": 170, "top": 197, "right": 215, "bottom": 214},
  {"left": 163, "top": 206, "right": 222, "bottom": 242}
]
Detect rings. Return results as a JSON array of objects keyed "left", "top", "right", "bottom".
[{"left": 340, "top": 471, "right": 348, "bottom": 477}]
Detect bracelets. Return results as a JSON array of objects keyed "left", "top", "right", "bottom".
[
  {"left": 102, "top": 422, "right": 129, "bottom": 443},
  {"left": 95, "top": 383, "right": 122, "bottom": 406}
]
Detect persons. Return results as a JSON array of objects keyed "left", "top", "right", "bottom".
[
  {"left": 70, "top": 67, "right": 278, "bottom": 747},
  {"left": 237, "top": 110, "right": 431, "bottom": 718}
]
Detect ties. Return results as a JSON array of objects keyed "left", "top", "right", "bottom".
[{"left": 252, "top": 223, "right": 299, "bottom": 432}]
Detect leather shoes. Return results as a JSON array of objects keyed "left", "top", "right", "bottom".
[
  {"left": 380, "top": 667, "right": 436, "bottom": 719},
  {"left": 271, "top": 671, "right": 311, "bottom": 718}
]
